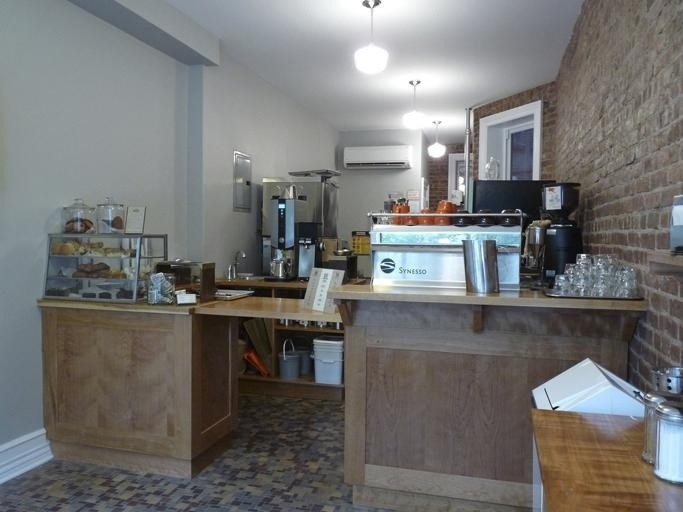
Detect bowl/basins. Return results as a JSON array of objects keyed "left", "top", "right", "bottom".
[{"left": 333, "top": 250, "right": 354, "bottom": 256}]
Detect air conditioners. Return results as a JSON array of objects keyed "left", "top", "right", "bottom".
[{"left": 342, "top": 145, "right": 412, "bottom": 170}]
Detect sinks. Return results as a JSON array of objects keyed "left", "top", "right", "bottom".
[{"left": 234, "top": 277, "right": 265, "bottom": 281}]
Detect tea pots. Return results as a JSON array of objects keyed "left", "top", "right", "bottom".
[{"left": 271, "top": 258, "right": 291, "bottom": 279}]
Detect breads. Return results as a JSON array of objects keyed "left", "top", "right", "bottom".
[
  {"left": 51, "top": 240, "right": 136, "bottom": 257},
  {"left": 70, "top": 263, "right": 127, "bottom": 279}
]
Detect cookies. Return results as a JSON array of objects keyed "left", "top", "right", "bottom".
[
  {"left": 64, "top": 217, "right": 93, "bottom": 233},
  {"left": 101, "top": 216, "right": 124, "bottom": 232}
]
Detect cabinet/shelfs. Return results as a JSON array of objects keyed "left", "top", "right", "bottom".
[
  {"left": 41, "top": 232, "right": 167, "bottom": 304},
  {"left": 213, "top": 278, "right": 367, "bottom": 403}
]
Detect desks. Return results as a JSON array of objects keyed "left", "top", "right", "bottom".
[{"left": 528, "top": 407, "right": 683, "bottom": 511}]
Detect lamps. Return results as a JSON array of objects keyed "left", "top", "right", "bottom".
[{"left": 351, "top": 0, "right": 446, "bottom": 160}]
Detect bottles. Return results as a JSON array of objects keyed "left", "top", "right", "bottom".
[
  {"left": 146, "top": 273, "right": 176, "bottom": 304},
  {"left": 642, "top": 392, "right": 683, "bottom": 484},
  {"left": 62, "top": 196, "right": 126, "bottom": 234}
]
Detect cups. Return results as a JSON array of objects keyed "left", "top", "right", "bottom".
[
  {"left": 418, "top": 200, "right": 456, "bottom": 225},
  {"left": 453, "top": 209, "right": 515, "bottom": 226},
  {"left": 553, "top": 253, "right": 638, "bottom": 299}
]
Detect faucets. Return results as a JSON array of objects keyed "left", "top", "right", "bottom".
[{"left": 231, "top": 249, "right": 246, "bottom": 278}]
